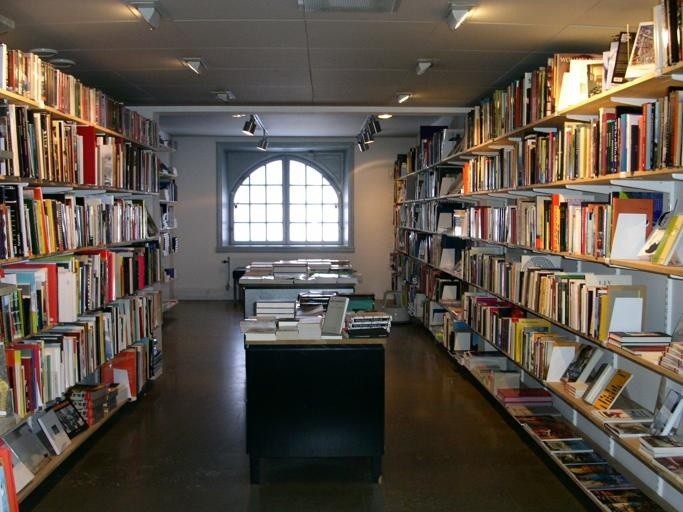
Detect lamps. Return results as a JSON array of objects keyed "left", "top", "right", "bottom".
[
  {"left": 444, "top": 2, "right": 476, "bottom": 32},
  {"left": 257, "top": 129, "right": 268, "bottom": 151},
  {"left": 132, "top": 7, "right": 160, "bottom": 30},
  {"left": 208, "top": 90, "right": 235, "bottom": 103},
  {"left": 182, "top": 57, "right": 207, "bottom": 74},
  {"left": 357, "top": 115, "right": 383, "bottom": 153},
  {"left": 413, "top": 58, "right": 434, "bottom": 77},
  {"left": 241, "top": 115, "right": 256, "bottom": 136},
  {"left": 395, "top": 91, "right": 412, "bottom": 104}
]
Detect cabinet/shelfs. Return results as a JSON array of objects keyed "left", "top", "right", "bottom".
[
  {"left": 244, "top": 330, "right": 385, "bottom": 483},
  {"left": 233, "top": 266, "right": 247, "bottom": 308},
  {"left": 394, "top": 61, "right": 683, "bottom": 512},
  {"left": 162, "top": 142, "right": 179, "bottom": 314},
  {"left": 239, "top": 274, "right": 357, "bottom": 320},
  {"left": 0, "top": 90, "right": 163, "bottom": 505}
]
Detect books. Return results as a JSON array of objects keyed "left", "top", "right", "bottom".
[
  {"left": 2, "top": 44, "right": 179, "bottom": 512},
  {"left": 391, "top": 0, "right": 683, "bottom": 512},
  {"left": 243, "top": 258, "right": 356, "bottom": 280},
  {"left": 239, "top": 291, "right": 392, "bottom": 339}
]
